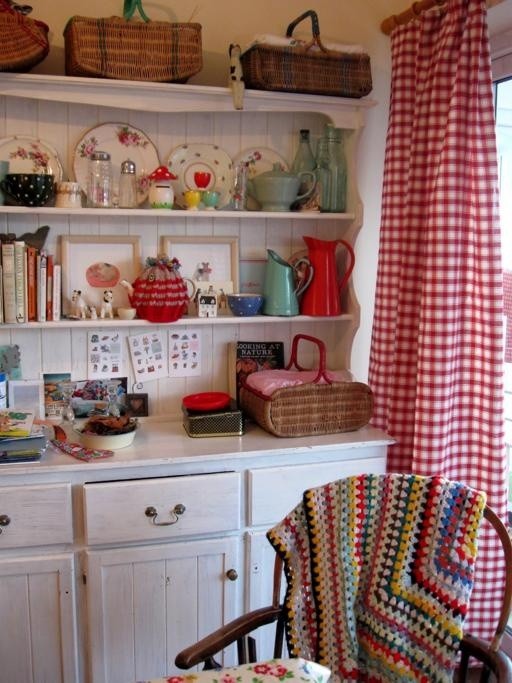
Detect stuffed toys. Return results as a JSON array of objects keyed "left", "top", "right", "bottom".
[
  {"left": 100, "top": 289, "right": 114, "bottom": 319},
  {"left": 87, "top": 305, "right": 98, "bottom": 319},
  {"left": 71, "top": 290, "right": 87, "bottom": 319}
]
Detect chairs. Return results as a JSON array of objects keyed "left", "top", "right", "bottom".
[{"left": 175, "top": 463, "right": 512, "bottom": 681}]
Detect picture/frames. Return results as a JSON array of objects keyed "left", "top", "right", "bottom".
[
  {"left": 56, "top": 232, "right": 143, "bottom": 318},
  {"left": 157, "top": 232, "right": 244, "bottom": 313}
]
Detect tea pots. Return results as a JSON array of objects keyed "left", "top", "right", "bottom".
[
  {"left": 120, "top": 258, "right": 199, "bottom": 322},
  {"left": 249, "top": 160, "right": 316, "bottom": 214}
]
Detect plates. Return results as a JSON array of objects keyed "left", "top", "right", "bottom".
[
  {"left": 73, "top": 122, "right": 288, "bottom": 210},
  {"left": 0, "top": 136, "right": 63, "bottom": 182}
]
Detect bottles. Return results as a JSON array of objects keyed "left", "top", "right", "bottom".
[
  {"left": 55, "top": 181, "right": 83, "bottom": 209},
  {"left": 295, "top": 120, "right": 349, "bottom": 214}
]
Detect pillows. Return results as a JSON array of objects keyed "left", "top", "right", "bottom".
[{"left": 116, "top": 657, "right": 335, "bottom": 681}]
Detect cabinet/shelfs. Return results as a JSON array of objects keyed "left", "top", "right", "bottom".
[{"left": 2, "top": 62, "right": 394, "bottom": 683}]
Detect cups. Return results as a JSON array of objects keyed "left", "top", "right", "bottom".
[
  {"left": 0, "top": 159, "right": 9, "bottom": 205},
  {"left": 1, "top": 173, "right": 55, "bottom": 206},
  {"left": 263, "top": 235, "right": 354, "bottom": 317}
]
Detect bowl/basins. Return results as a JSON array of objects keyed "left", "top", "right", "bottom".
[
  {"left": 181, "top": 392, "right": 233, "bottom": 410},
  {"left": 227, "top": 293, "right": 263, "bottom": 315},
  {"left": 117, "top": 307, "right": 136, "bottom": 321},
  {"left": 71, "top": 417, "right": 137, "bottom": 452}
]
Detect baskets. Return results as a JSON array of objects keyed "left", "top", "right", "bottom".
[
  {"left": 62, "top": 0, "right": 204, "bottom": 82},
  {"left": 0, "top": 0, "right": 50, "bottom": 72},
  {"left": 237, "top": 9, "right": 374, "bottom": 100},
  {"left": 238, "top": 333, "right": 375, "bottom": 438}
]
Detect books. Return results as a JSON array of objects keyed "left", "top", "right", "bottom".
[
  {"left": 226, "top": 339, "right": 284, "bottom": 401},
  {"left": 0, "top": 238, "right": 62, "bottom": 324},
  {"left": 1, "top": 407, "right": 36, "bottom": 437}
]
